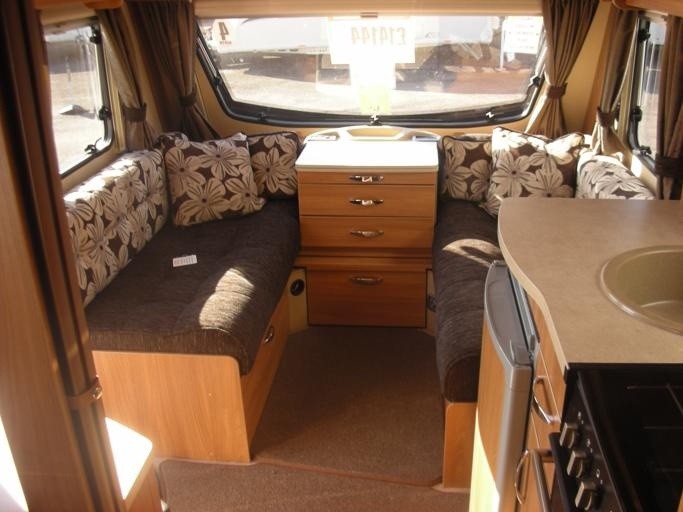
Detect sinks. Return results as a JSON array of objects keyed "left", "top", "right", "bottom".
[{"left": 601, "top": 246, "right": 682, "bottom": 333}]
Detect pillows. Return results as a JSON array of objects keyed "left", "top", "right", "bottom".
[
  {"left": 159, "top": 132, "right": 266, "bottom": 227},
  {"left": 478, "top": 126, "right": 584, "bottom": 220}
]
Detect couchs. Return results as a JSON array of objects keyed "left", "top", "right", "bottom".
[
  {"left": 427, "top": 133, "right": 657, "bottom": 488},
  {"left": 62, "top": 130, "right": 308, "bottom": 464}
]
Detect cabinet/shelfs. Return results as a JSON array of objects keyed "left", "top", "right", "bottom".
[
  {"left": 469, "top": 196, "right": 682, "bottom": 512},
  {"left": 293, "top": 139, "right": 440, "bottom": 328}
]
